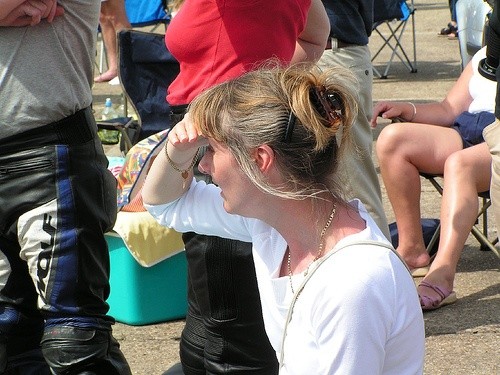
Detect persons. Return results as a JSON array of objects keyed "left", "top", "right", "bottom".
[
  {"left": 0, "top": 0, "right": 132, "bottom": 375},
  {"left": 163, "top": 0, "right": 331, "bottom": 375},
  {"left": 94, "top": 0, "right": 500, "bottom": 311},
  {"left": 141, "top": 56, "right": 425, "bottom": 375}
]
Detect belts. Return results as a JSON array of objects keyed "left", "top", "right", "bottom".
[{"left": 325, "top": 37, "right": 349, "bottom": 49}]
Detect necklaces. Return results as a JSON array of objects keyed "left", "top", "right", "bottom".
[{"left": 287, "top": 201, "right": 336, "bottom": 297}]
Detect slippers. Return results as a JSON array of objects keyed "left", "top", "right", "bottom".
[
  {"left": 406, "top": 262, "right": 430, "bottom": 276},
  {"left": 417, "top": 281, "right": 457, "bottom": 309}
]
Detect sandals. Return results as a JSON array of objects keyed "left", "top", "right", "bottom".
[{"left": 438, "top": 22, "right": 459, "bottom": 40}]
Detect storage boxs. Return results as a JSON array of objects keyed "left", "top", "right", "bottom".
[{"left": 104, "top": 231, "right": 188, "bottom": 326}]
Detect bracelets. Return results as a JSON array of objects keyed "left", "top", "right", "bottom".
[
  {"left": 408, "top": 102, "right": 417, "bottom": 122},
  {"left": 164, "top": 139, "right": 200, "bottom": 179}
]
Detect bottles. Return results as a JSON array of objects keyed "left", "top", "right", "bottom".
[{"left": 102, "top": 98, "right": 117, "bottom": 121}]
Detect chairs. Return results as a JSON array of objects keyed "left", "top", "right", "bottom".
[
  {"left": 371, "top": 0, "right": 418, "bottom": 79},
  {"left": 377, "top": 112, "right": 500, "bottom": 261},
  {"left": 95, "top": 0, "right": 171, "bottom": 74}
]
